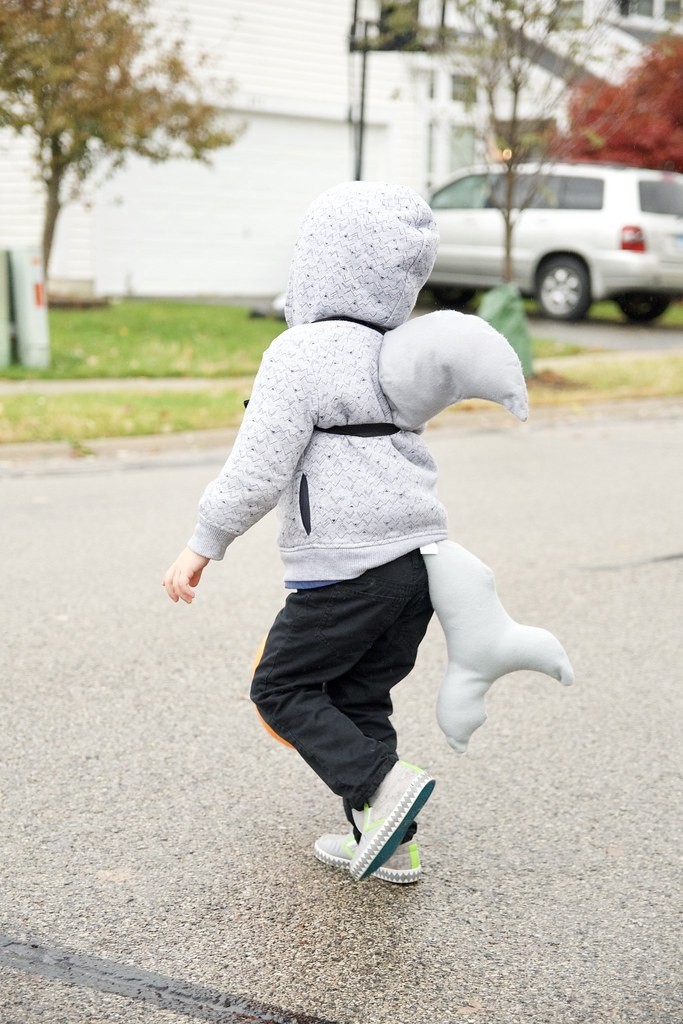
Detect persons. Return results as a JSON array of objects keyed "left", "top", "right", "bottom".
[{"left": 159, "top": 179, "right": 452, "bottom": 884}]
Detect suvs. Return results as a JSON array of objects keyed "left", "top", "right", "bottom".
[{"left": 416, "top": 162, "right": 683, "bottom": 321}]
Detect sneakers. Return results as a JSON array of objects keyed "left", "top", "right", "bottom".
[
  {"left": 315, "top": 831, "right": 421, "bottom": 885},
  {"left": 349, "top": 762, "right": 435, "bottom": 882}
]
